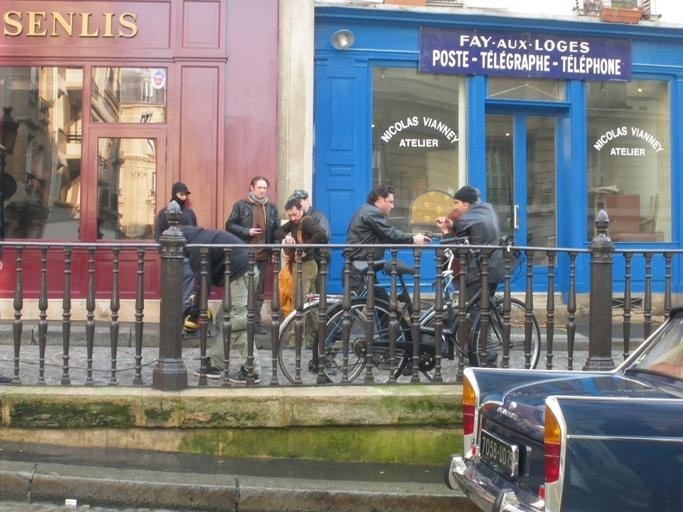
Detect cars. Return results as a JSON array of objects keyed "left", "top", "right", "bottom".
[{"left": 443, "top": 310, "right": 681, "bottom": 511}]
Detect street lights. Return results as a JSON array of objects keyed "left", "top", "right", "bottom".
[{"left": 1, "top": 106, "right": 18, "bottom": 240}]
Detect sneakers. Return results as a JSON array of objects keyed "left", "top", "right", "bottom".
[
  {"left": 228, "top": 369, "right": 260, "bottom": 383},
  {"left": 194, "top": 366, "right": 221, "bottom": 379}
]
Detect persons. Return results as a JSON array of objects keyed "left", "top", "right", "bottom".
[
  {"left": 435, "top": 186, "right": 506, "bottom": 367},
  {"left": 442, "top": 186, "right": 482, "bottom": 290},
  {"left": 309, "top": 184, "right": 433, "bottom": 375},
  {"left": 154, "top": 176, "right": 331, "bottom": 383}
]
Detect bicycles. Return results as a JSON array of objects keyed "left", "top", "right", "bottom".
[{"left": 278, "top": 236, "right": 542, "bottom": 385}]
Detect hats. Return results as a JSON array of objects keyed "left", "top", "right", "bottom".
[
  {"left": 291, "top": 191, "right": 307, "bottom": 200},
  {"left": 172, "top": 184, "right": 191, "bottom": 194},
  {"left": 454, "top": 186, "right": 477, "bottom": 203}
]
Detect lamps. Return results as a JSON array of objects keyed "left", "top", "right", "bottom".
[{"left": 330, "top": 29, "right": 355, "bottom": 52}]
